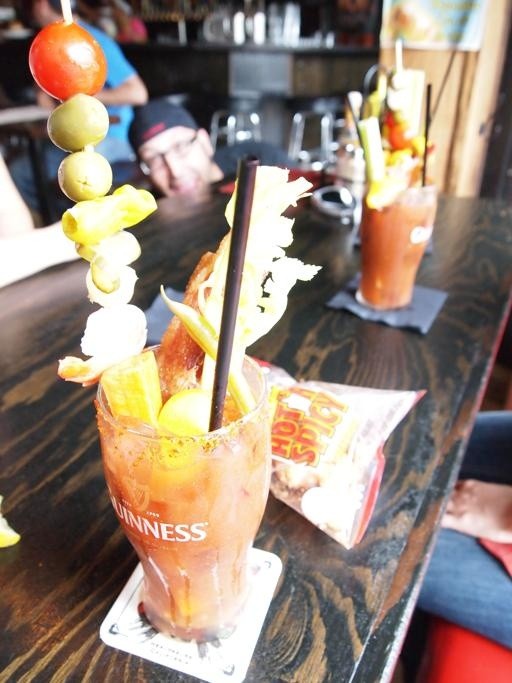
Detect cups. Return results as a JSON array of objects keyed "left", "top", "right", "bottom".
[
  {"left": 94, "top": 340, "right": 275, "bottom": 643},
  {"left": 355, "top": 181, "right": 439, "bottom": 311}
]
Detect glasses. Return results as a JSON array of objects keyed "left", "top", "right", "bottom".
[{"left": 140, "top": 130, "right": 199, "bottom": 176}]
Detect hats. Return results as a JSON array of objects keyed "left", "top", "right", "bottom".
[{"left": 128, "top": 101, "right": 199, "bottom": 152}]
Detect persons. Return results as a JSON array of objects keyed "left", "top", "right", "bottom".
[
  {"left": 395, "top": 410, "right": 512, "bottom": 682},
  {"left": 129, "top": 102, "right": 303, "bottom": 198},
  {"left": 109, "top": 1, "right": 147, "bottom": 45},
  {"left": 11, "top": 1, "right": 150, "bottom": 227}
]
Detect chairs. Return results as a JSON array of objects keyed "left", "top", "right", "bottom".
[
  {"left": 60, "top": 87, "right": 353, "bottom": 202},
  {"left": 414, "top": 546, "right": 512, "bottom": 683}
]
